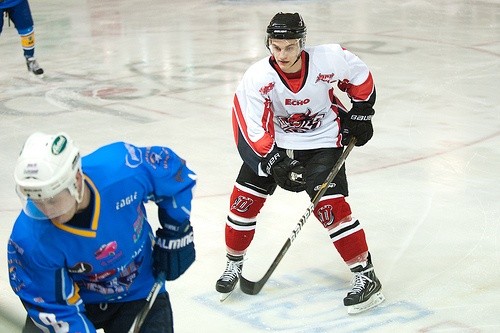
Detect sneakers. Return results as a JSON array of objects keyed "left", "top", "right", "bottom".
[
  {"left": 26, "top": 57, "right": 44, "bottom": 78},
  {"left": 343, "top": 250, "right": 385, "bottom": 314},
  {"left": 216, "top": 256, "right": 246, "bottom": 302}
]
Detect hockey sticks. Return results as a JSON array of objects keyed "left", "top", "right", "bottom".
[
  {"left": 127, "top": 267, "right": 168, "bottom": 333},
  {"left": 240, "top": 138, "right": 357, "bottom": 295}
]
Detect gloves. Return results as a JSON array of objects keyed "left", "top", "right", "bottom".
[
  {"left": 152, "top": 225, "right": 195, "bottom": 280},
  {"left": 261, "top": 150, "right": 309, "bottom": 192},
  {"left": 341, "top": 103, "right": 375, "bottom": 147}
]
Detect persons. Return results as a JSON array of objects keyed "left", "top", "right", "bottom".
[
  {"left": 0, "top": 0, "right": 47, "bottom": 74},
  {"left": 7, "top": 132, "right": 198, "bottom": 333},
  {"left": 214, "top": 11, "right": 382, "bottom": 307}
]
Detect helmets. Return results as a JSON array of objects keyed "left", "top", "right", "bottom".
[
  {"left": 265, "top": 12, "right": 308, "bottom": 39},
  {"left": 14, "top": 130, "right": 86, "bottom": 205}
]
